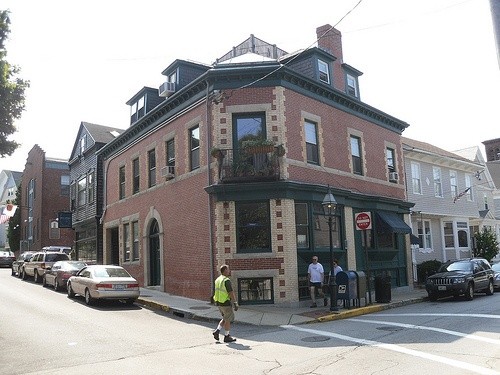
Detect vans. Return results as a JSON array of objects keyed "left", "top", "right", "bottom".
[{"left": 42, "top": 246, "right": 73, "bottom": 260}]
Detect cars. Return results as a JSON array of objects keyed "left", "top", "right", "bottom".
[
  {"left": 490, "top": 261, "right": 500, "bottom": 289},
  {"left": 11, "top": 252, "right": 35, "bottom": 278},
  {"left": 42, "top": 261, "right": 88, "bottom": 292},
  {"left": 0, "top": 251, "right": 16, "bottom": 269},
  {"left": 67, "top": 265, "right": 140, "bottom": 306}
]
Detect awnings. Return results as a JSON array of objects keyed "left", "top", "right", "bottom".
[{"left": 376, "top": 211, "right": 411, "bottom": 234}]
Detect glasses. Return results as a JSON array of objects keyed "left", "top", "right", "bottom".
[{"left": 312, "top": 259, "right": 317, "bottom": 261}]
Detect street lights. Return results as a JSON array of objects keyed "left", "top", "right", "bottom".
[{"left": 320, "top": 184, "right": 339, "bottom": 312}]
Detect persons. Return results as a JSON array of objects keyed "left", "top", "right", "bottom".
[
  {"left": 212, "top": 265, "right": 238, "bottom": 342},
  {"left": 307, "top": 256, "right": 328, "bottom": 308},
  {"left": 329, "top": 258, "right": 343, "bottom": 276}
]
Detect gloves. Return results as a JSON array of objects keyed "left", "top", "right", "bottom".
[{"left": 233, "top": 303, "right": 239, "bottom": 311}]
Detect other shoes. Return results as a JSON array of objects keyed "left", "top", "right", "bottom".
[
  {"left": 324, "top": 299, "right": 327, "bottom": 306},
  {"left": 310, "top": 302, "right": 317, "bottom": 308}
]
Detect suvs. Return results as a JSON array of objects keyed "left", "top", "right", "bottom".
[
  {"left": 424, "top": 257, "right": 495, "bottom": 302},
  {"left": 22, "top": 252, "right": 70, "bottom": 284}
]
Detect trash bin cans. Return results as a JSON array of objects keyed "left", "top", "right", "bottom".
[
  {"left": 350, "top": 270, "right": 367, "bottom": 307},
  {"left": 375, "top": 275, "right": 391, "bottom": 303},
  {"left": 335, "top": 271, "right": 357, "bottom": 308}
]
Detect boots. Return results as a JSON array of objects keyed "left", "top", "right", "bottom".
[
  {"left": 212, "top": 329, "right": 220, "bottom": 340},
  {"left": 224, "top": 334, "right": 236, "bottom": 342}
]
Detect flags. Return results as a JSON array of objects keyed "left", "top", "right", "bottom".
[
  {"left": 3, "top": 204, "right": 17, "bottom": 217},
  {"left": 0, "top": 215, "right": 10, "bottom": 224}
]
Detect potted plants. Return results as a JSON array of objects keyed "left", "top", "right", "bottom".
[
  {"left": 241, "top": 136, "right": 275, "bottom": 153},
  {"left": 231, "top": 160, "right": 278, "bottom": 177},
  {"left": 210, "top": 147, "right": 221, "bottom": 159},
  {"left": 278, "top": 143, "right": 286, "bottom": 157}
]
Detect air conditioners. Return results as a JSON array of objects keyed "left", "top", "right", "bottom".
[
  {"left": 162, "top": 166, "right": 176, "bottom": 177},
  {"left": 389, "top": 172, "right": 399, "bottom": 183},
  {"left": 159, "top": 82, "right": 175, "bottom": 98}
]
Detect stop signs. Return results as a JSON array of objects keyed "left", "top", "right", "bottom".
[{"left": 355, "top": 213, "right": 370, "bottom": 229}]
